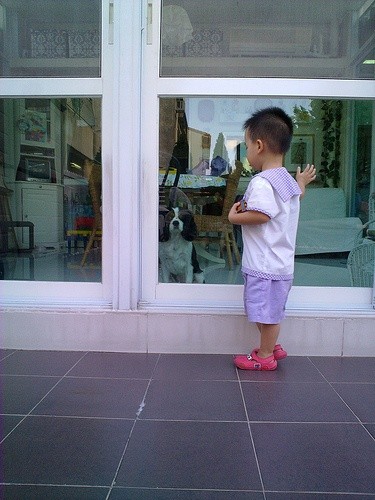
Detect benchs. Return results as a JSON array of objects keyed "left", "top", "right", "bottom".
[{"left": 295, "top": 188, "right": 363, "bottom": 256}]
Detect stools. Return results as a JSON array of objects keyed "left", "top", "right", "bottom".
[{"left": 67, "top": 230, "right": 101, "bottom": 249}]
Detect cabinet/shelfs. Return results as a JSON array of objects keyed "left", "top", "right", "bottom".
[{"left": 22, "top": 188, "right": 58, "bottom": 243}]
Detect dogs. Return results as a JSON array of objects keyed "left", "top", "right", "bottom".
[{"left": 159, "top": 206, "right": 206, "bottom": 283}]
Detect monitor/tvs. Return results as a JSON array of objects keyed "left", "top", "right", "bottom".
[{"left": 19, "top": 158, "right": 52, "bottom": 182}]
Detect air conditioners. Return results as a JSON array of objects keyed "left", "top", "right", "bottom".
[{"left": 228, "top": 26, "right": 312, "bottom": 56}]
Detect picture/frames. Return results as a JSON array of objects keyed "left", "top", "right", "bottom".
[{"left": 283, "top": 134, "right": 315, "bottom": 173}]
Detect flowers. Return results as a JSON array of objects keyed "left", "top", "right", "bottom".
[{"left": 16, "top": 110, "right": 44, "bottom": 141}]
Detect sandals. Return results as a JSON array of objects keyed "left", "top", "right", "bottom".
[
  {"left": 234, "top": 348, "right": 277, "bottom": 370},
  {"left": 273, "top": 344, "right": 287, "bottom": 359}
]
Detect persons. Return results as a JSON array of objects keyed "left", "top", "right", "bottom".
[{"left": 228, "top": 107, "right": 316, "bottom": 372}]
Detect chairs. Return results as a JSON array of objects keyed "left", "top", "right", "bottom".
[
  {"left": 159, "top": 152, "right": 181, "bottom": 215},
  {"left": 347, "top": 241, "right": 375, "bottom": 287},
  {"left": 79, "top": 160, "right": 102, "bottom": 268},
  {"left": 191, "top": 160, "right": 243, "bottom": 271}
]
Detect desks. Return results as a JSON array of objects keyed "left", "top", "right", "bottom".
[{"left": 159, "top": 169, "right": 226, "bottom": 196}]
